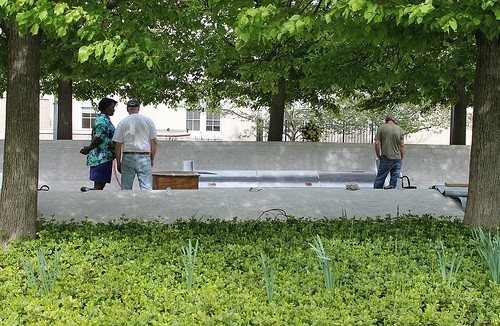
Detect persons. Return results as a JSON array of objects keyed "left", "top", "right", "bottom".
[
  {"left": 373, "top": 115, "right": 404, "bottom": 189},
  {"left": 79, "top": 98, "right": 118, "bottom": 190},
  {"left": 112, "top": 99, "right": 158, "bottom": 191}
]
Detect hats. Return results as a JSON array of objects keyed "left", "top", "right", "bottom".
[{"left": 127, "top": 100, "right": 139, "bottom": 107}]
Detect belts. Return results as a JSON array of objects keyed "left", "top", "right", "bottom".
[{"left": 123, "top": 152, "right": 151, "bottom": 155}]
